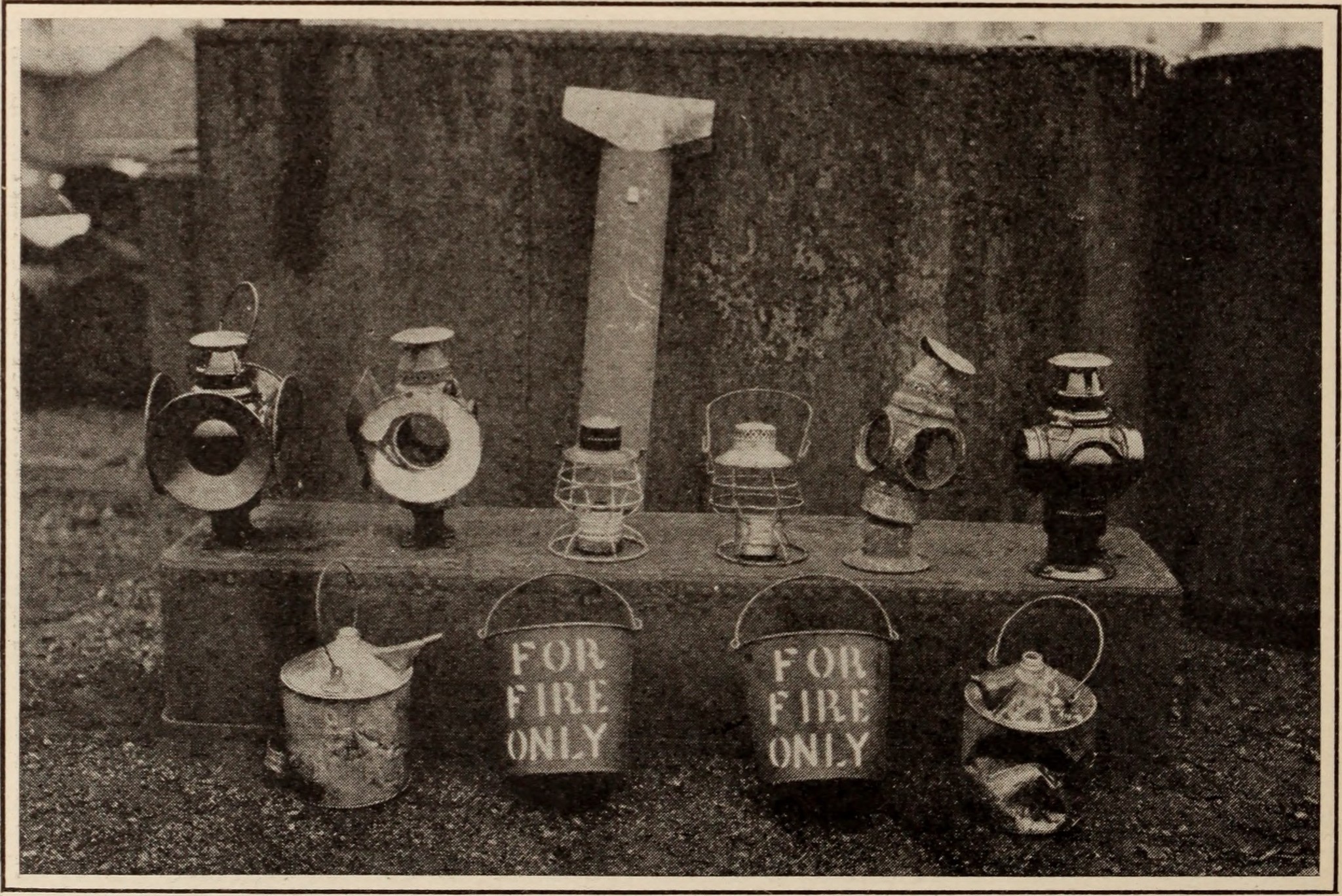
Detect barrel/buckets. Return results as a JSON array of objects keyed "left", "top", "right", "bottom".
[
  {"left": 726, "top": 572, "right": 902, "bottom": 788},
  {"left": 474, "top": 572, "right": 645, "bottom": 780}
]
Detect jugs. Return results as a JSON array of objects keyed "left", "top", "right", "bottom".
[
  {"left": 264, "top": 559, "right": 443, "bottom": 810},
  {"left": 957, "top": 591, "right": 1106, "bottom": 838}
]
